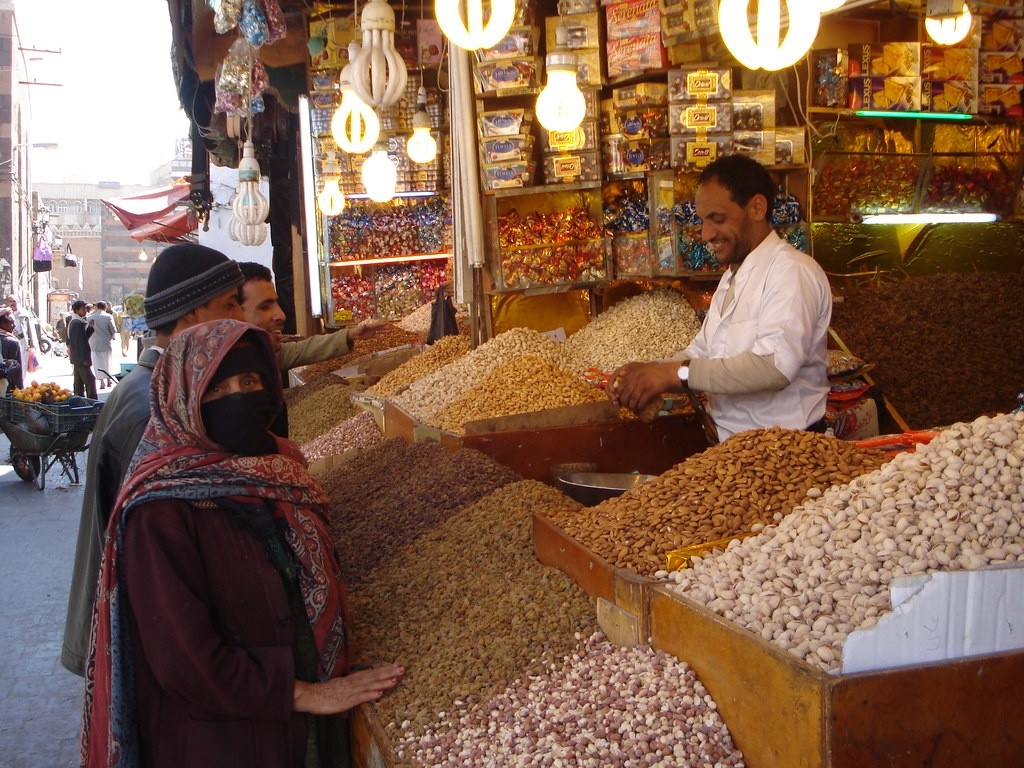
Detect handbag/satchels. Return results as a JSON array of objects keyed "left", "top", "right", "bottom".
[{"left": 27, "top": 346, "right": 42, "bottom": 372}]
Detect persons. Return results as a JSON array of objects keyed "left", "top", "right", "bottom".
[
  {"left": 68, "top": 300, "right": 98, "bottom": 400},
  {"left": 604, "top": 154, "right": 832, "bottom": 443},
  {"left": 59, "top": 242, "right": 390, "bottom": 683},
  {"left": 88, "top": 301, "right": 117, "bottom": 389},
  {"left": 119, "top": 306, "right": 130, "bottom": 356},
  {"left": 55, "top": 301, "right": 117, "bottom": 341},
  {"left": 79, "top": 319, "right": 402, "bottom": 768},
  {"left": 0, "top": 294, "right": 36, "bottom": 397}
]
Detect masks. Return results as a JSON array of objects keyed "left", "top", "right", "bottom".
[{"left": 200, "top": 388, "right": 280, "bottom": 450}]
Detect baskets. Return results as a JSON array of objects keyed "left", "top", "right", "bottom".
[{"left": 0, "top": 381, "right": 107, "bottom": 433}]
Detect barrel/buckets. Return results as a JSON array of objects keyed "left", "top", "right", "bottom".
[{"left": 119, "top": 363, "right": 139, "bottom": 376}]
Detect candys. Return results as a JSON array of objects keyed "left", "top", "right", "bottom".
[
  {"left": 214, "top": 0, "right": 289, "bottom": 115},
  {"left": 311, "top": 69, "right": 807, "bottom": 325},
  {"left": 811, "top": 163, "right": 1024, "bottom": 219}
]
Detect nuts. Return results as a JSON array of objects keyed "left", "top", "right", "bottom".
[{"left": 281, "top": 269, "right": 1024, "bottom": 768}]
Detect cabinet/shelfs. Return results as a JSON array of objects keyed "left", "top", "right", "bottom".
[{"left": 309, "top": 0, "right": 1024, "bottom": 331}]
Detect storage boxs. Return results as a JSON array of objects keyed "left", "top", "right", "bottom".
[{"left": 0, "top": 395, "right": 105, "bottom": 435}]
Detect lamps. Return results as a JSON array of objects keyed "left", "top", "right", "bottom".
[
  {"left": 849, "top": 110, "right": 978, "bottom": 119},
  {"left": 850, "top": 212, "right": 1001, "bottom": 224},
  {"left": 296, "top": 93, "right": 322, "bottom": 319}
]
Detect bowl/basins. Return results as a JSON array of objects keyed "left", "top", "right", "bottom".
[{"left": 557, "top": 472, "right": 658, "bottom": 508}]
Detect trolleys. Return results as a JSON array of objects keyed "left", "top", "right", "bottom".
[{"left": 0, "top": 394, "right": 106, "bottom": 490}]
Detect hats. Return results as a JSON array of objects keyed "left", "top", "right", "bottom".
[
  {"left": 144, "top": 243, "right": 246, "bottom": 330},
  {"left": 208, "top": 330, "right": 273, "bottom": 386}
]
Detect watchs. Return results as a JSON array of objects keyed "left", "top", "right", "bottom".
[{"left": 677, "top": 359, "right": 691, "bottom": 389}]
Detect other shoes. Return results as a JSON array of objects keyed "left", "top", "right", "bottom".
[
  {"left": 107, "top": 381, "right": 112, "bottom": 387},
  {"left": 122, "top": 352, "right": 127, "bottom": 357},
  {"left": 100, "top": 382, "right": 106, "bottom": 389}
]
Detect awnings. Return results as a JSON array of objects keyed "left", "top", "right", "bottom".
[{"left": 100, "top": 179, "right": 198, "bottom": 241}]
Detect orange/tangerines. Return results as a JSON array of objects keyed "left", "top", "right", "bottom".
[{"left": 13, "top": 379, "right": 72, "bottom": 407}]
